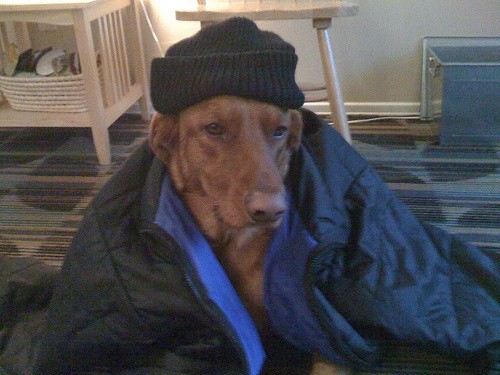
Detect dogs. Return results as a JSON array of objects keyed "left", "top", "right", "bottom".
[{"left": 148, "top": 92, "right": 351, "bottom": 375}]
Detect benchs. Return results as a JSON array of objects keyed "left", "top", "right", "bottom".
[{"left": 420, "top": 35, "right": 500, "bottom": 146}]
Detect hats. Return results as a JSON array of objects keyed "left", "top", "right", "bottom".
[{"left": 146, "top": 14, "right": 304, "bottom": 110}]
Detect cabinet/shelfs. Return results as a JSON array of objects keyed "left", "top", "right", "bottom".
[{"left": 0, "top": 1, "right": 151, "bottom": 166}]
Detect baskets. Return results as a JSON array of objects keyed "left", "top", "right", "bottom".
[{"left": 0, "top": 65, "right": 111, "bottom": 112}]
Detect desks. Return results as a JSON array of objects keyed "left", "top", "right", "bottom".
[{"left": 174, "top": 1, "right": 359, "bottom": 154}]
